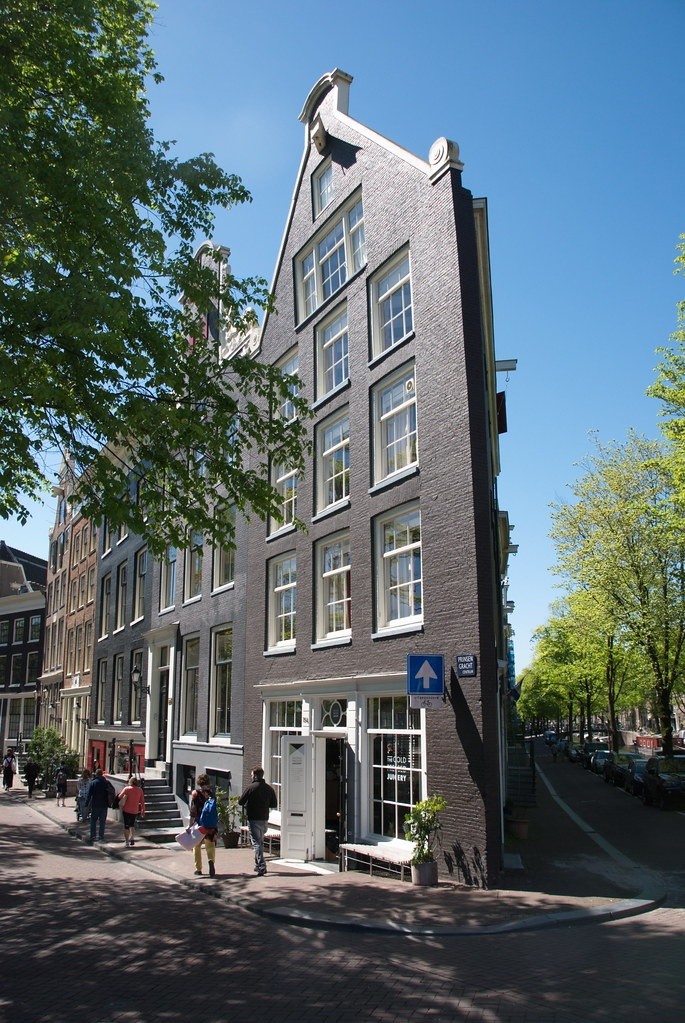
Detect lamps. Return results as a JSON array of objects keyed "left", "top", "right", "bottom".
[
  {"left": 42, "top": 685, "right": 49, "bottom": 692},
  {"left": 33, "top": 686, "right": 38, "bottom": 693},
  {"left": 76, "top": 700, "right": 82, "bottom": 709},
  {"left": 48, "top": 704, "right": 55, "bottom": 709},
  {"left": 130, "top": 665, "right": 150, "bottom": 695}
]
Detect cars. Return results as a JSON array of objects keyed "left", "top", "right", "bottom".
[{"left": 543, "top": 730, "right": 685, "bottom": 812}]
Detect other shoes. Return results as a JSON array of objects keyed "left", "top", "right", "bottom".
[
  {"left": 256, "top": 869, "right": 267, "bottom": 876},
  {"left": 56, "top": 804, "right": 60, "bottom": 807},
  {"left": 209, "top": 860, "right": 216, "bottom": 877},
  {"left": 88, "top": 836, "right": 96, "bottom": 841},
  {"left": 62, "top": 803, "right": 66, "bottom": 807},
  {"left": 99, "top": 838, "right": 106, "bottom": 843},
  {"left": 5, "top": 785, "right": 9, "bottom": 791},
  {"left": 125, "top": 841, "right": 130, "bottom": 848},
  {"left": 130, "top": 839, "right": 135, "bottom": 845},
  {"left": 28, "top": 794, "right": 32, "bottom": 798},
  {"left": 194, "top": 870, "right": 202, "bottom": 875},
  {"left": 254, "top": 867, "right": 259, "bottom": 871}
]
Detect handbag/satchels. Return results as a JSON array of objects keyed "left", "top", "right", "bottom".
[{"left": 118, "top": 788, "right": 129, "bottom": 808}]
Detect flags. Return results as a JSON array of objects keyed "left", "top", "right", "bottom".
[{"left": 511, "top": 681, "right": 522, "bottom": 702}]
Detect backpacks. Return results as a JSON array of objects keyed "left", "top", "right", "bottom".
[
  {"left": 56, "top": 769, "right": 67, "bottom": 787},
  {"left": 195, "top": 789, "right": 218, "bottom": 830},
  {"left": 106, "top": 781, "right": 115, "bottom": 806}
]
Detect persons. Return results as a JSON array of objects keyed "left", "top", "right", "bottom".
[
  {"left": 85, "top": 769, "right": 108, "bottom": 841},
  {"left": 77, "top": 770, "right": 92, "bottom": 823},
  {"left": 185, "top": 774, "right": 216, "bottom": 876},
  {"left": 55, "top": 761, "right": 67, "bottom": 807},
  {"left": 93, "top": 761, "right": 100, "bottom": 773},
  {"left": 24, "top": 757, "right": 36, "bottom": 798},
  {"left": 3, "top": 748, "right": 15, "bottom": 791},
  {"left": 238, "top": 768, "right": 277, "bottom": 876},
  {"left": 118, "top": 778, "right": 145, "bottom": 847}
]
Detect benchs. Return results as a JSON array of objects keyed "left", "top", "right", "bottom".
[
  {"left": 239, "top": 808, "right": 281, "bottom": 856},
  {"left": 340, "top": 832, "right": 426, "bottom": 881}
]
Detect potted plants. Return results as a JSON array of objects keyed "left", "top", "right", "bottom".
[
  {"left": 401, "top": 792, "right": 447, "bottom": 886},
  {"left": 214, "top": 785, "right": 243, "bottom": 848},
  {"left": 42, "top": 749, "right": 58, "bottom": 798}
]
